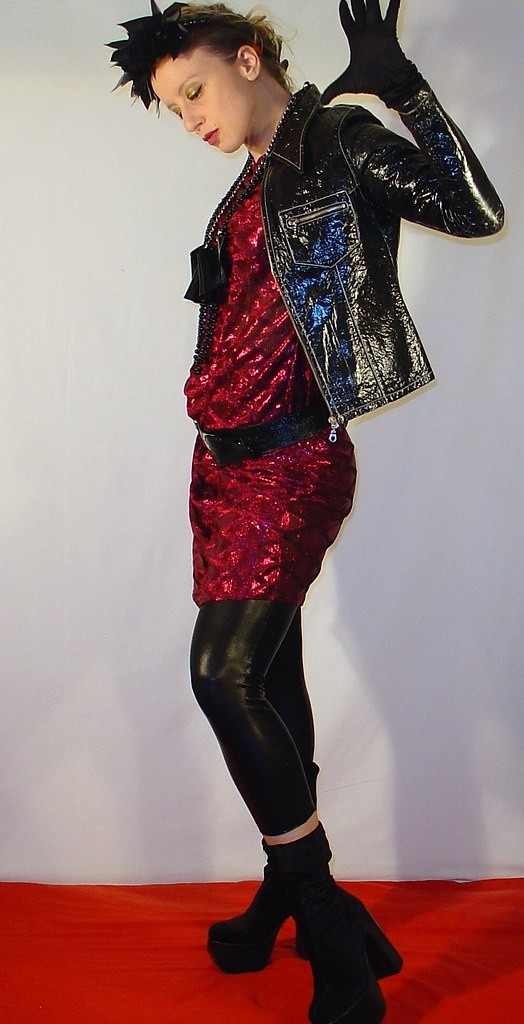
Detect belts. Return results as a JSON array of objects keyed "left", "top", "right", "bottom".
[{"left": 192, "top": 398, "right": 330, "bottom": 467}]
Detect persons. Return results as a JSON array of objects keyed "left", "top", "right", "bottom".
[{"left": 106, "top": 0, "right": 504, "bottom": 1024}]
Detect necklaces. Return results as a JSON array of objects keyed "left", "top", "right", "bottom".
[{"left": 193, "top": 96, "right": 296, "bottom": 373}]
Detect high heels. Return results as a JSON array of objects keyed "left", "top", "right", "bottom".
[
  {"left": 293, "top": 876, "right": 403, "bottom": 1024},
  {"left": 206, "top": 867, "right": 295, "bottom": 974}
]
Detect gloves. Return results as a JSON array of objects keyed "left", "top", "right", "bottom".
[{"left": 319, "top": 0, "right": 426, "bottom": 110}]
